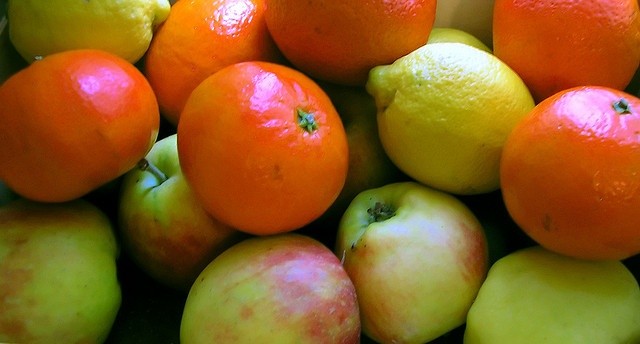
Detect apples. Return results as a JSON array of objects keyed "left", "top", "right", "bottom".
[
  {"left": 322, "top": 82, "right": 393, "bottom": 215},
  {"left": 174, "top": 234, "right": 364, "bottom": 344},
  {"left": 464, "top": 249, "right": 640, "bottom": 342},
  {"left": 2, "top": 194, "right": 121, "bottom": 342},
  {"left": 123, "top": 135, "right": 234, "bottom": 288}
]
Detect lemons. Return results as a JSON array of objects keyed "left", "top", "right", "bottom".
[
  {"left": 7, "top": 1, "right": 169, "bottom": 66},
  {"left": 362, "top": 44, "right": 534, "bottom": 193}
]
